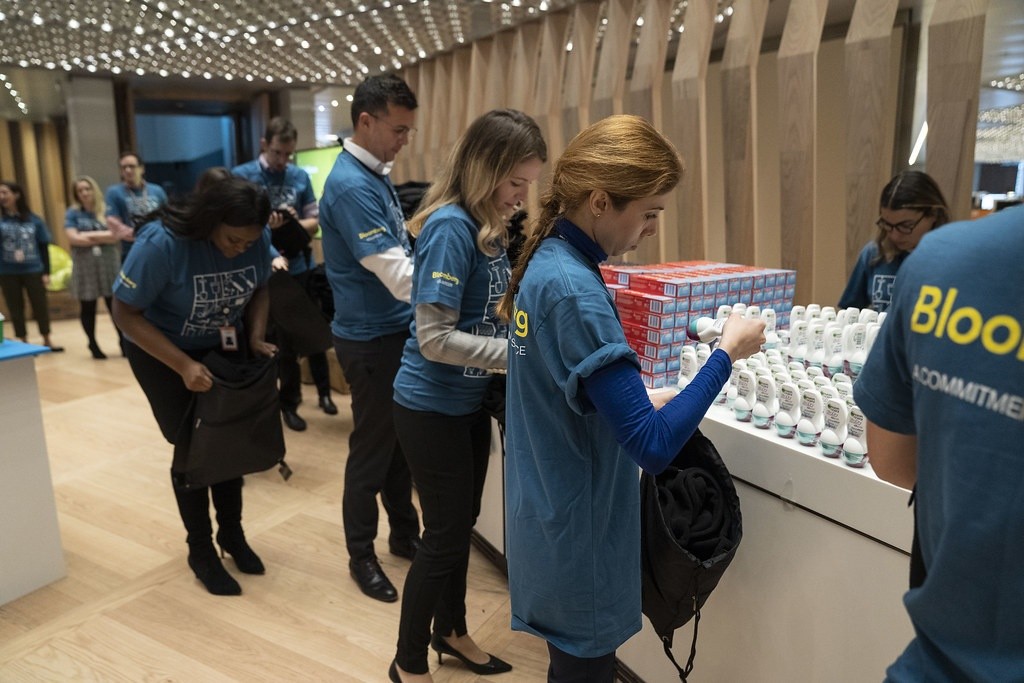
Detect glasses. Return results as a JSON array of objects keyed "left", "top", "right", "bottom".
[
  {"left": 264, "top": 139, "right": 297, "bottom": 161},
  {"left": 362, "top": 111, "right": 419, "bottom": 141},
  {"left": 874, "top": 211, "right": 930, "bottom": 235},
  {"left": 121, "top": 163, "right": 142, "bottom": 171}
]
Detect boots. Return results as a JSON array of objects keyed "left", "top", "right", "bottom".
[
  {"left": 211, "top": 477, "right": 265, "bottom": 576},
  {"left": 168, "top": 466, "right": 241, "bottom": 596}
]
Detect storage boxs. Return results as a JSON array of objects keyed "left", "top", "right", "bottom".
[
  {"left": 297, "top": 357, "right": 315, "bottom": 385},
  {"left": 325, "top": 347, "right": 353, "bottom": 395},
  {"left": 600, "top": 260, "right": 796, "bottom": 390}
]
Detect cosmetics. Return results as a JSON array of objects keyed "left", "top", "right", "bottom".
[{"left": 679, "top": 304, "right": 887, "bottom": 470}]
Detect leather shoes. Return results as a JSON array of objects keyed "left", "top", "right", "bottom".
[
  {"left": 389, "top": 531, "right": 424, "bottom": 561},
  {"left": 348, "top": 556, "right": 398, "bottom": 603}
]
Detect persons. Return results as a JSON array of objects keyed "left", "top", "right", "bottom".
[
  {"left": 105, "top": 151, "right": 168, "bottom": 262},
  {"left": 834, "top": 171, "right": 948, "bottom": 310},
  {"left": 389, "top": 106, "right": 546, "bottom": 683},
  {"left": 507, "top": 116, "right": 763, "bottom": 683},
  {"left": 226, "top": 337, "right": 233, "bottom": 345},
  {"left": 0, "top": 182, "right": 64, "bottom": 352},
  {"left": 63, "top": 175, "right": 124, "bottom": 357},
  {"left": 233, "top": 116, "right": 337, "bottom": 431},
  {"left": 111, "top": 169, "right": 280, "bottom": 596},
  {"left": 851, "top": 200, "right": 1024, "bottom": 683},
  {"left": 319, "top": 75, "right": 419, "bottom": 604}
]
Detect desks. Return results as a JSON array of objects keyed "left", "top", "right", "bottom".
[
  {"left": 0, "top": 339, "right": 68, "bottom": 609},
  {"left": 471, "top": 385, "right": 918, "bottom": 683}
]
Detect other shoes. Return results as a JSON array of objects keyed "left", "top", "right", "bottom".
[
  {"left": 44, "top": 344, "right": 64, "bottom": 351},
  {"left": 89, "top": 343, "right": 106, "bottom": 358},
  {"left": 319, "top": 394, "right": 337, "bottom": 415},
  {"left": 281, "top": 409, "right": 307, "bottom": 431}
]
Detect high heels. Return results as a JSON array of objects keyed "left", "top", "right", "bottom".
[
  {"left": 388, "top": 658, "right": 402, "bottom": 683},
  {"left": 430, "top": 632, "right": 513, "bottom": 675}
]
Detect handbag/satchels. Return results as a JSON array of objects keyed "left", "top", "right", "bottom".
[
  {"left": 182, "top": 362, "right": 286, "bottom": 492},
  {"left": 618, "top": 424, "right": 744, "bottom": 649}
]
